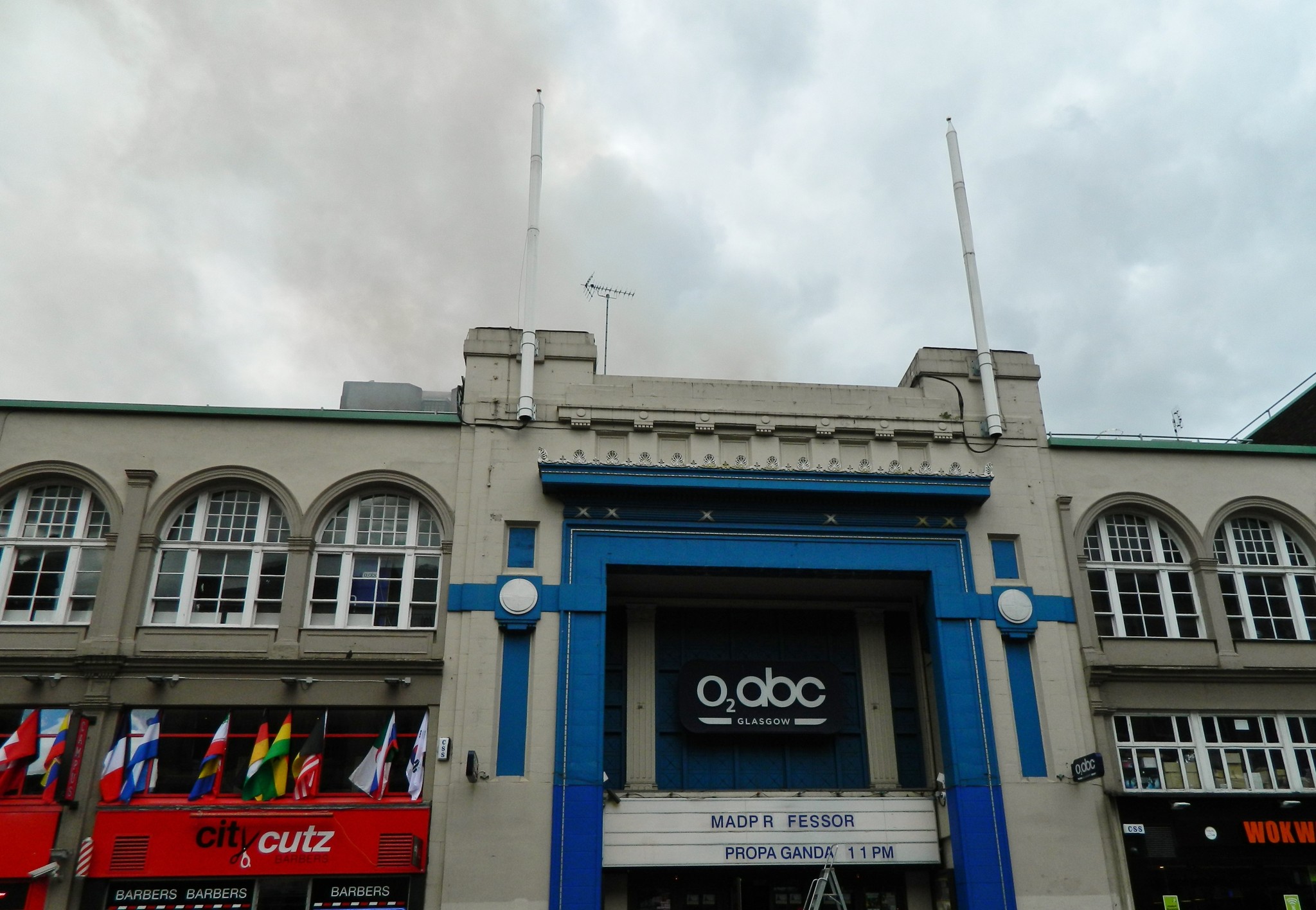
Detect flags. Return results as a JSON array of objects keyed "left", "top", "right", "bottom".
[
  {"left": 348, "top": 708, "right": 398, "bottom": 800},
  {"left": 241, "top": 709, "right": 271, "bottom": 801},
  {"left": 41, "top": 710, "right": 73, "bottom": 802},
  {"left": 188, "top": 713, "right": 231, "bottom": 802},
  {"left": 0, "top": 707, "right": 43, "bottom": 796},
  {"left": 292, "top": 710, "right": 328, "bottom": 800},
  {"left": 404, "top": 710, "right": 430, "bottom": 802},
  {"left": 99, "top": 717, "right": 132, "bottom": 801},
  {"left": 120, "top": 713, "right": 164, "bottom": 803},
  {"left": 257, "top": 709, "right": 293, "bottom": 802}
]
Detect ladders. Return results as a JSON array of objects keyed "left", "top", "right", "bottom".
[{"left": 802, "top": 844, "right": 848, "bottom": 910}]
